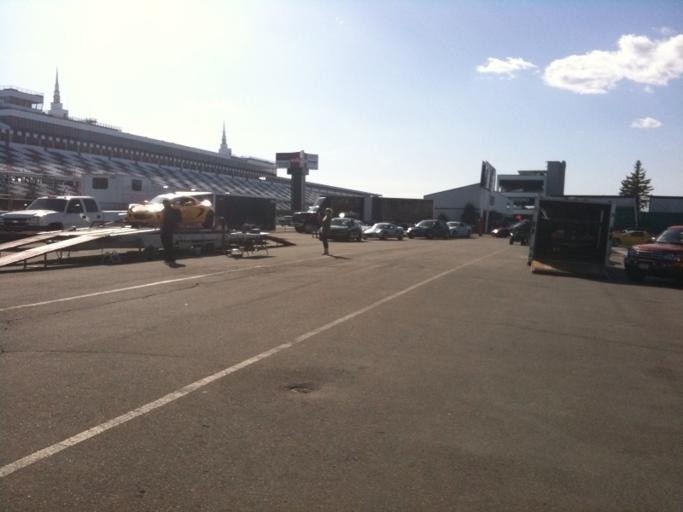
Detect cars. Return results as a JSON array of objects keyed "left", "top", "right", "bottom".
[
  {"left": 319, "top": 216, "right": 531, "bottom": 249},
  {"left": 609, "top": 228, "right": 655, "bottom": 248}
]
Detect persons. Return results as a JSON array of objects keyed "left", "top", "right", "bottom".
[
  {"left": 319, "top": 208, "right": 333, "bottom": 255},
  {"left": 160, "top": 198, "right": 178, "bottom": 266}
]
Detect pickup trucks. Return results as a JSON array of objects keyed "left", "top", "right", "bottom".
[{"left": 0, "top": 194, "right": 129, "bottom": 231}]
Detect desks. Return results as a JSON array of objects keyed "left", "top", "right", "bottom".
[{"left": 227, "top": 231, "right": 268, "bottom": 256}]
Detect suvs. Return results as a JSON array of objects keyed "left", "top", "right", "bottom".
[
  {"left": 624, "top": 224, "right": 682, "bottom": 282},
  {"left": 278, "top": 214, "right": 292, "bottom": 227}
]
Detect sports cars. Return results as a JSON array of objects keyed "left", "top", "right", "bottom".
[{"left": 126, "top": 191, "right": 215, "bottom": 229}]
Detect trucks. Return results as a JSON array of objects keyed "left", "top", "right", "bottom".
[
  {"left": 173, "top": 188, "right": 277, "bottom": 233},
  {"left": 292, "top": 195, "right": 433, "bottom": 233}
]
